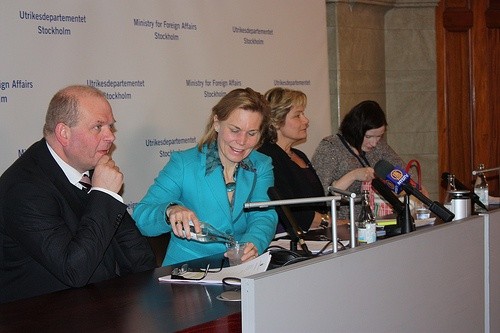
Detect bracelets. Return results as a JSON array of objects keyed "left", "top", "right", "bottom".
[{"left": 165, "top": 203, "right": 178, "bottom": 224}]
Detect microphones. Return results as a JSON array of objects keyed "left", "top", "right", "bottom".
[
  {"left": 441, "top": 171, "right": 486, "bottom": 210},
  {"left": 371, "top": 177, "right": 414, "bottom": 221},
  {"left": 373, "top": 160, "right": 456, "bottom": 224},
  {"left": 267, "top": 187, "right": 310, "bottom": 257}
]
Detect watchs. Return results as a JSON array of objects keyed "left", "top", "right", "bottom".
[{"left": 320, "top": 214, "right": 329, "bottom": 228}]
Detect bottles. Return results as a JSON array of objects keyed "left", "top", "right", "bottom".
[
  {"left": 175, "top": 220, "right": 234, "bottom": 243},
  {"left": 356, "top": 190, "right": 376, "bottom": 246},
  {"left": 443, "top": 174, "right": 456, "bottom": 212},
  {"left": 474, "top": 163, "right": 489, "bottom": 215}
]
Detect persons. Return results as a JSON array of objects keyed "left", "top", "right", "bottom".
[
  {"left": 0, "top": 85, "right": 156, "bottom": 304},
  {"left": 310, "top": 100, "right": 428, "bottom": 220},
  {"left": 256, "top": 87, "right": 348, "bottom": 239},
  {"left": 132, "top": 88, "right": 278, "bottom": 266}
]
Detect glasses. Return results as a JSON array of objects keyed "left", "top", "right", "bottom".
[{"left": 171, "top": 258, "right": 225, "bottom": 281}]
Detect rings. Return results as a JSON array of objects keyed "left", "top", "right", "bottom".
[{"left": 175, "top": 220, "right": 183, "bottom": 226}]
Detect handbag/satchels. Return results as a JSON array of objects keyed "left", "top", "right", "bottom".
[{"left": 361, "top": 159, "right": 430, "bottom": 218}]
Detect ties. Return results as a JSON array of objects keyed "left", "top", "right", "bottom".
[{"left": 79, "top": 174, "right": 91, "bottom": 189}]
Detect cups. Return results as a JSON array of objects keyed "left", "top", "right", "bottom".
[
  {"left": 414, "top": 200, "right": 431, "bottom": 220},
  {"left": 225, "top": 241, "right": 248, "bottom": 267}
]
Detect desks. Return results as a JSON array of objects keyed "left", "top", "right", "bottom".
[{"left": 0, "top": 207, "right": 500, "bottom": 333}]
